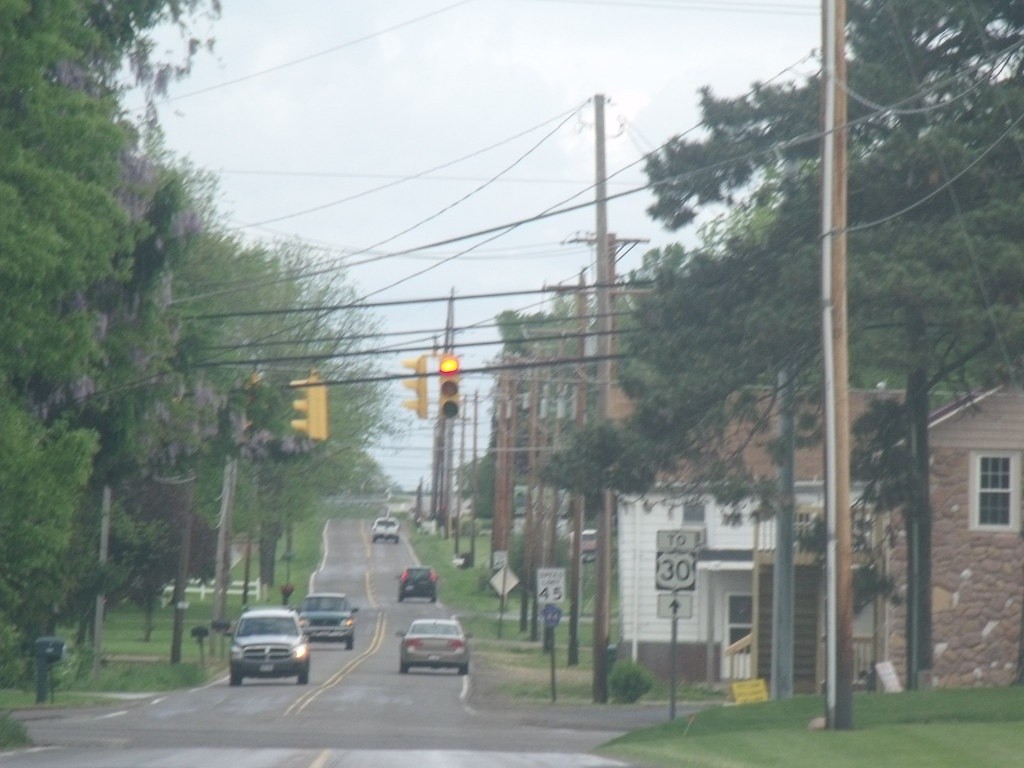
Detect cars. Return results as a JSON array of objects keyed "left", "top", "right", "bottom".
[
  {"left": 372, "top": 517, "right": 400, "bottom": 543},
  {"left": 396, "top": 568, "right": 438, "bottom": 602},
  {"left": 299, "top": 593, "right": 358, "bottom": 649},
  {"left": 223, "top": 608, "right": 310, "bottom": 685},
  {"left": 396, "top": 619, "right": 472, "bottom": 674}
]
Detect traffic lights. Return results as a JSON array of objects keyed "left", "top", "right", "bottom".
[
  {"left": 289, "top": 370, "right": 329, "bottom": 441},
  {"left": 439, "top": 356, "right": 460, "bottom": 417},
  {"left": 403, "top": 356, "right": 427, "bottom": 417}
]
